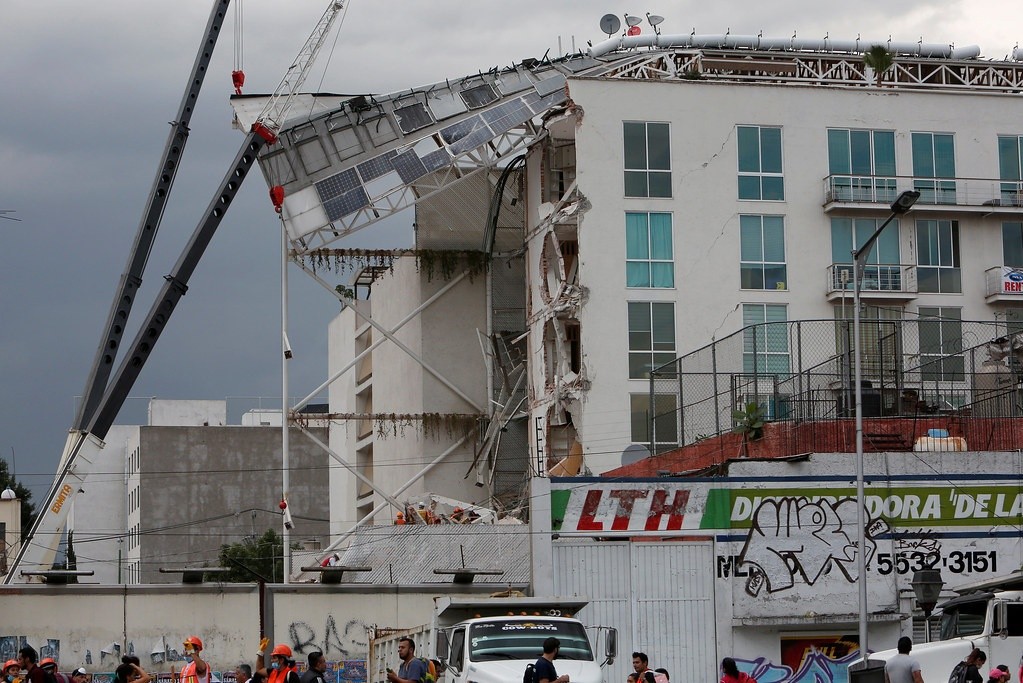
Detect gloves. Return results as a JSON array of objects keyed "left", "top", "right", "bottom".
[
  {"left": 257, "top": 638, "right": 270, "bottom": 656},
  {"left": 183, "top": 638, "right": 196, "bottom": 655}
]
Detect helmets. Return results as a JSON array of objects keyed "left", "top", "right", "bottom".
[
  {"left": 334, "top": 553, "right": 341, "bottom": 560},
  {"left": 183, "top": 636, "right": 202, "bottom": 651},
  {"left": 419, "top": 502, "right": 425, "bottom": 506},
  {"left": 396, "top": 512, "right": 403, "bottom": 517},
  {"left": 270, "top": 645, "right": 292, "bottom": 657},
  {"left": 40, "top": 658, "right": 58, "bottom": 672},
  {"left": 2, "top": 660, "right": 20, "bottom": 673}
]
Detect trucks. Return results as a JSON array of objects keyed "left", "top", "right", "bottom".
[
  {"left": 366, "top": 595, "right": 619, "bottom": 683},
  {"left": 847, "top": 571, "right": 1023, "bottom": 683}
]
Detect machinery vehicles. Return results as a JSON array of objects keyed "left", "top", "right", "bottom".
[{"left": 5, "top": 1, "right": 347, "bottom": 580}]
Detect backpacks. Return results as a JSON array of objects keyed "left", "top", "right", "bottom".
[
  {"left": 949, "top": 663, "right": 977, "bottom": 683},
  {"left": 645, "top": 670, "right": 669, "bottom": 683},
  {"left": 524, "top": 664, "right": 536, "bottom": 683}
]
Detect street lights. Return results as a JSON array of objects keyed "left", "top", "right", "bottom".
[
  {"left": 850, "top": 191, "right": 921, "bottom": 657},
  {"left": 909, "top": 568, "right": 947, "bottom": 643}
]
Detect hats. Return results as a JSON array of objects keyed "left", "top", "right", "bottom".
[
  {"left": 989, "top": 669, "right": 1007, "bottom": 679},
  {"left": 78, "top": 668, "right": 87, "bottom": 674}
]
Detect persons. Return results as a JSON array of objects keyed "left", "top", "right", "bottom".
[
  {"left": 387, "top": 638, "right": 426, "bottom": 683},
  {"left": 393, "top": 500, "right": 465, "bottom": 525},
  {"left": 958, "top": 648, "right": 1011, "bottom": 683},
  {"left": 627, "top": 673, "right": 639, "bottom": 683},
  {"left": 632, "top": 651, "right": 656, "bottom": 683},
  {"left": 535, "top": 637, "right": 569, "bottom": 683},
  {"left": 884, "top": 636, "right": 924, "bottom": 683},
  {"left": 719, "top": 657, "right": 757, "bottom": 683},
  {"left": 319, "top": 552, "right": 341, "bottom": 567},
  {"left": 0, "top": 637, "right": 327, "bottom": 683},
  {"left": 655, "top": 667, "right": 671, "bottom": 683}
]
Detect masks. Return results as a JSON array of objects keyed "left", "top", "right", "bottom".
[
  {"left": 553, "top": 648, "right": 558, "bottom": 660},
  {"left": 976, "top": 664, "right": 982, "bottom": 669},
  {"left": 272, "top": 659, "right": 282, "bottom": 669},
  {"left": 184, "top": 657, "right": 193, "bottom": 662}
]
